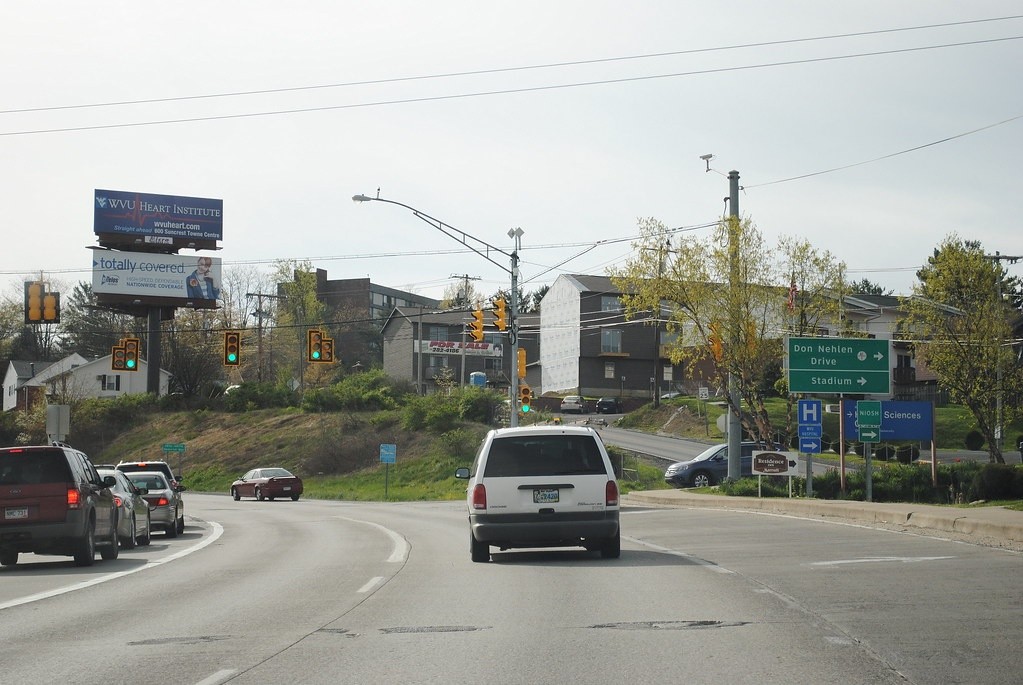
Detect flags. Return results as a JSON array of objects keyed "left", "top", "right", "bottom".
[{"left": 788, "top": 271, "right": 798, "bottom": 315}]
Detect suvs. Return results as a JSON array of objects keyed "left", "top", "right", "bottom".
[
  {"left": 0, "top": 441, "right": 119, "bottom": 567},
  {"left": 115, "top": 458, "right": 183, "bottom": 498},
  {"left": 454, "top": 425, "right": 621, "bottom": 561},
  {"left": 664, "top": 441, "right": 789, "bottom": 488}
]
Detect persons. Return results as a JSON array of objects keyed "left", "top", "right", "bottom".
[
  {"left": 493, "top": 344, "right": 502, "bottom": 357},
  {"left": 186, "top": 257, "right": 220, "bottom": 299}
]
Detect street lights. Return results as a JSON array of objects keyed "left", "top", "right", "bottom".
[{"left": 353, "top": 194, "right": 519, "bottom": 425}]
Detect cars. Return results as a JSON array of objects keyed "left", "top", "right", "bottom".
[
  {"left": 661, "top": 393, "right": 684, "bottom": 399},
  {"left": 596, "top": 398, "right": 624, "bottom": 414},
  {"left": 230, "top": 466, "right": 303, "bottom": 502},
  {"left": 560, "top": 396, "right": 590, "bottom": 413},
  {"left": 96, "top": 470, "right": 150, "bottom": 549},
  {"left": 123, "top": 471, "right": 186, "bottom": 537}
]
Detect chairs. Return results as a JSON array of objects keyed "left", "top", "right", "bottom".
[
  {"left": 560, "top": 449, "right": 584, "bottom": 467},
  {"left": 147, "top": 480, "right": 158, "bottom": 489}
]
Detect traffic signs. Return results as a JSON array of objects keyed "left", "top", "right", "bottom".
[{"left": 788, "top": 337, "right": 891, "bottom": 394}]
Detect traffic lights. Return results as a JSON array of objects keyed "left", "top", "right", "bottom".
[
  {"left": 224, "top": 332, "right": 241, "bottom": 366},
  {"left": 307, "top": 329, "right": 334, "bottom": 363},
  {"left": 468, "top": 311, "right": 484, "bottom": 342},
  {"left": 111, "top": 338, "right": 139, "bottom": 371},
  {"left": 492, "top": 299, "right": 505, "bottom": 330},
  {"left": 521, "top": 386, "right": 531, "bottom": 413}
]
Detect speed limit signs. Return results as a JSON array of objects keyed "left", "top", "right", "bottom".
[{"left": 699, "top": 387, "right": 708, "bottom": 399}]
